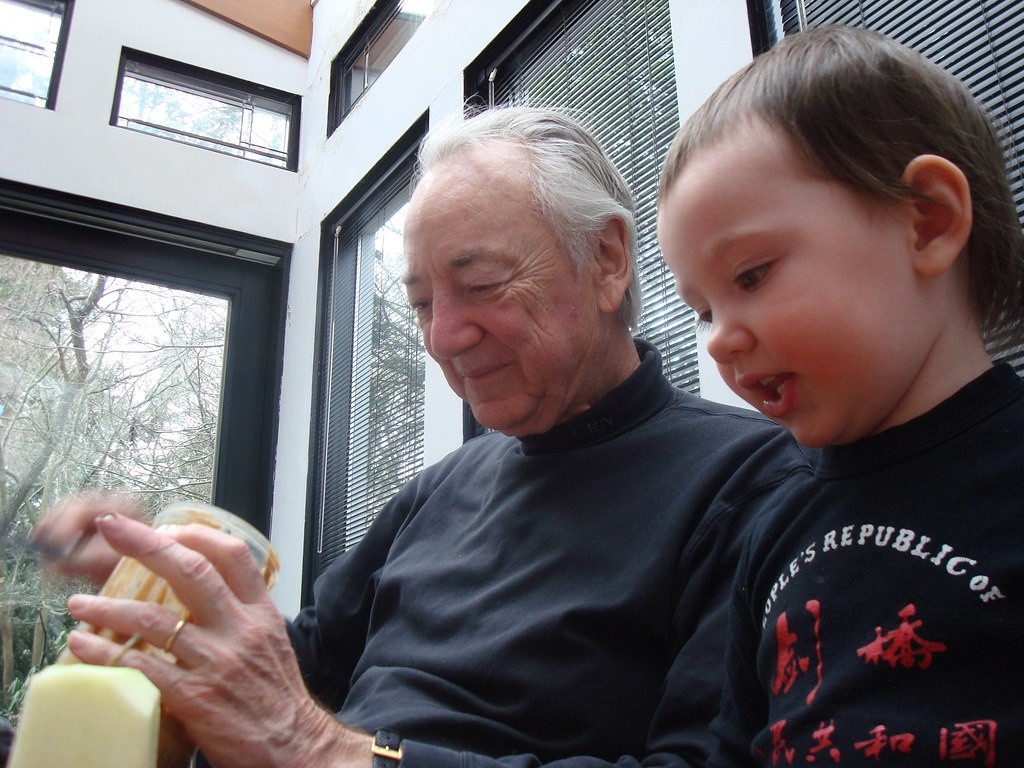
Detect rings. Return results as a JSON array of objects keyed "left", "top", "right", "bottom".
[{"left": 163, "top": 614, "right": 189, "bottom": 656}]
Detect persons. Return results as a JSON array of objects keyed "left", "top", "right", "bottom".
[
  {"left": 656, "top": 25, "right": 1024, "bottom": 768},
  {"left": 34, "top": 107, "right": 805, "bottom": 768}
]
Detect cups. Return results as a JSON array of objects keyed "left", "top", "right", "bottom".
[{"left": 55, "top": 499, "right": 279, "bottom": 768}]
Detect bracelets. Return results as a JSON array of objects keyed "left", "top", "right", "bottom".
[{"left": 371, "top": 724, "right": 404, "bottom": 767}]
